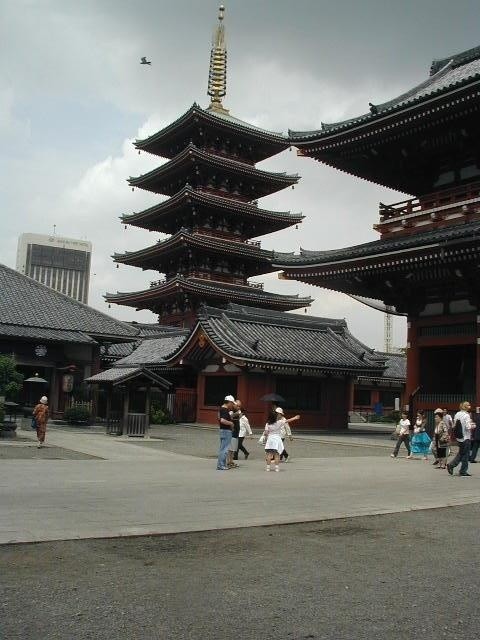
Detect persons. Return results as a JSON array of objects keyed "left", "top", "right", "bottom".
[
  {"left": 391, "top": 401, "right": 480, "bottom": 476},
  {"left": 217, "top": 395, "right": 301, "bottom": 472},
  {"left": 33, "top": 395, "right": 50, "bottom": 448}
]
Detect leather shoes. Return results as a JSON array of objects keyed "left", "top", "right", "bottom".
[
  {"left": 447, "top": 464, "right": 453, "bottom": 475},
  {"left": 461, "top": 473, "right": 471, "bottom": 476}
]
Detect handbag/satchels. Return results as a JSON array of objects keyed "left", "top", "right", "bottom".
[{"left": 258, "top": 432, "right": 266, "bottom": 446}]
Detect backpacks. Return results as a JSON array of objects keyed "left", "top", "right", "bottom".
[{"left": 454, "top": 419, "right": 463, "bottom": 439}]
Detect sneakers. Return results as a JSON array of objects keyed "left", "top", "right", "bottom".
[{"left": 217, "top": 463, "right": 239, "bottom": 470}]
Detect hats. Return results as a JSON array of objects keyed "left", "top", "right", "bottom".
[
  {"left": 225, "top": 395, "right": 237, "bottom": 405},
  {"left": 275, "top": 408, "right": 284, "bottom": 416}
]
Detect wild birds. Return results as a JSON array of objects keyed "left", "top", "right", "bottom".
[{"left": 141, "top": 57, "right": 151, "bottom": 65}]
